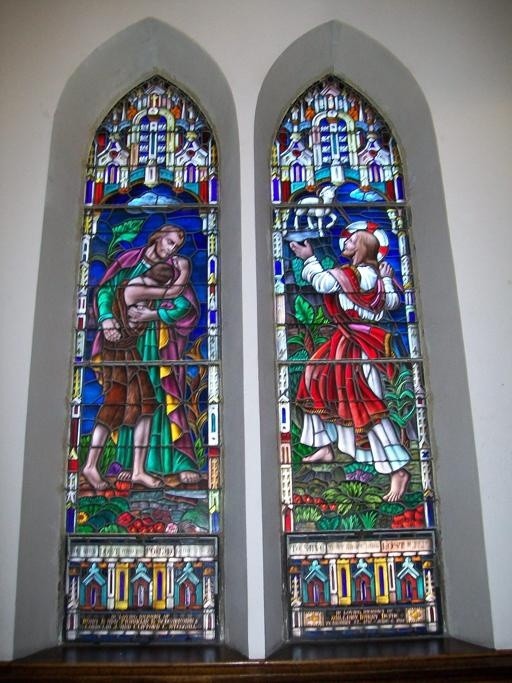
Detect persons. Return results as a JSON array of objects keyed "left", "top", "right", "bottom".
[
  {"left": 92, "top": 225, "right": 204, "bottom": 485},
  {"left": 80, "top": 256, "right": 191, "bottom": 490},
  {"left": 290, "top": 229, "right": 412, "bottom": 502}
]
